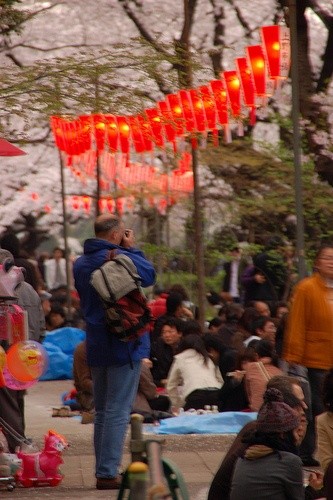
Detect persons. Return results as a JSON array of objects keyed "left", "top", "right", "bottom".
[
  {"left": 231, "top": 388, "right": 324, "bottom": 500},
  {"left": 315, "top": 369, "right": 333, "bottom": 474},
  {"left": 0, "top": 231, "right": 333, "bottom": 454},
  {"left": 284, "top": 243, "right": 332, "bottom": 468},
  {"left": 73, "top": 214, "right": 155, "bottom": 491},
  {"left": 207, "top": 375, "right": 308, "bottom": 500}
]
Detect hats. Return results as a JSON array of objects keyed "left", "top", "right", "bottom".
[{"left": 255, "top": 388, "right": 299, "bottom": 433}]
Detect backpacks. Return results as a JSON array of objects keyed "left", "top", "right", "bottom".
[{"left": 90, "top": 254, "right": 151, "bottom": 344}]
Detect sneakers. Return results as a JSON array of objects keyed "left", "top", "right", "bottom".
[{"left": 96, "top": 479, "right": 129, "bottom": 489}]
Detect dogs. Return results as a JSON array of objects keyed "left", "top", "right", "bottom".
[{"left": 0, "top": 429, "right": 71, "bottom": 491}]
